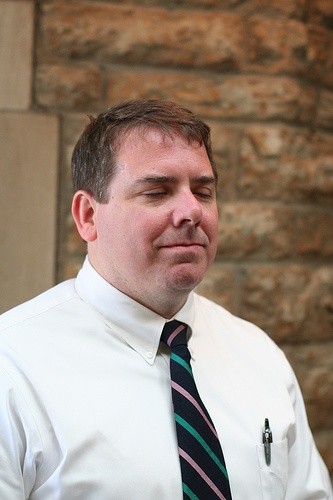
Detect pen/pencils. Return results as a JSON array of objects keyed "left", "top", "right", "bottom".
[{"left": 262, "top": 419, "right": 273, "bottom": 466}]
